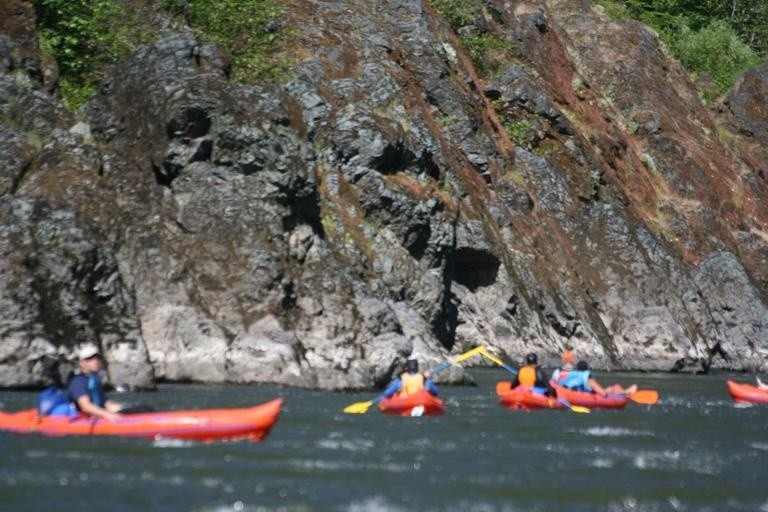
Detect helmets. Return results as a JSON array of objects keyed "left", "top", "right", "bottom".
[
  {"left": 564, "top": 354, "right": 572, "bottom": 362},
  {"left": 407, "top": 360, "right": 418, "bottom": 371},
  {"left": 526, "top": 354, "right": 538, "bottom": 364},
  {"left": 576, "top": 361, "right": 587, "bottom": 369}
]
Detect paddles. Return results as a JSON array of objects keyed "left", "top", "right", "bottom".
[
  {"left": 479, "top": 348, "right": 590, "bottom": 414},
  {"left": 343, "top": 345, "right": 484, "bottom": 414},
  {"left": 612, "top": 389, "right": 658, "bottom": 405}
]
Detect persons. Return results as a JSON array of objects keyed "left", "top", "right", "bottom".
[
  {"left": 510, "top": 353, "right": 558, "bottom": 397},
  {"left": 67, "top": 340, "right": 155, "bottom": 420},
  {"left": 550, "top": 349, "right": 576, "bottom": 383},
  {"left": 755, "top": 376, "right": 767, "bottom": 391},
  {"left": 381, "top": 358, "right": 437, "bottom": 400},
  {"left": 564, "top": 359, "right": 638, "bottom": 396}
]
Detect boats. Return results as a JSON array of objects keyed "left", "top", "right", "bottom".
[
  {"left": 380, "top": 392, "right": 445, "bottom": 417},
  {"left": 0, "top": 399, "right": 284, "bottom": 445},
  {"left": 728, "top": 379, "right": 766, "bottom": 404},
  {"left": 495, "top": 379, "right": 631, "bottom": 409}
]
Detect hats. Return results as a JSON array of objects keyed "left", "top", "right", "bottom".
[{"left": 79, "top": 346, "right": 102, "bottom": 359}]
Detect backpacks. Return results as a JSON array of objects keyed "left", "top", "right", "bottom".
[{"left": 38, "top": 387, "right": 77, "bottom": 416}]
